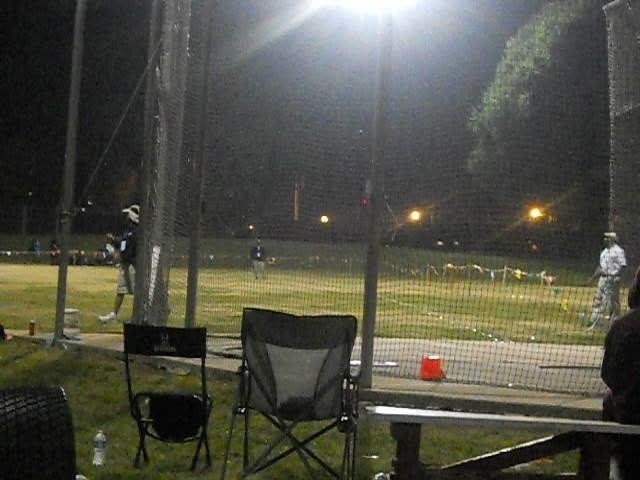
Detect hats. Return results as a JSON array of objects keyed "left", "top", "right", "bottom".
[
  {"left": 122, "top": 205, "right": 140, "bottom": 223},
  {"left": 602, "top": 231, "right": 619, "bottom": 243}
]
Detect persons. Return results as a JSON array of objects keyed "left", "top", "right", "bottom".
[
  {"left": 599, "top": 265, "right": 640, "bottom": 480},
  {"left": 31, "top": 238, "right": 90, "bottom": 267},
  {"left": 97, "top": 203, "right": 141, "bottom": 324},
  {"left": 0, "top": 323, "right": 13, "bottom": 341},
  {"left": 584, "top": 230, "right": 627, "bottom": 336},
  {"left": 249, "top": 239, "right": 269, "bottom": 281}
]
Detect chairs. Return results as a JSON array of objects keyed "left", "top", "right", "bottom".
[
  {"left": 120, "top": 319, "right": 216, "bottom": 471},
  {"left": 220, "top": 306, "right": 366, "bottom": 480}
]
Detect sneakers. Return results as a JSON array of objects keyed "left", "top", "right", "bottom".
[{"left": 99, "top": 312, "right": 116, "bottom": 324}]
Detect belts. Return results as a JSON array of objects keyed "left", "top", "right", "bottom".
[{"left": 599, "top": 273, "right": 614, "bottom": 277}]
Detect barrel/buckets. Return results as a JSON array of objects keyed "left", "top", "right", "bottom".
[
  {"left": 63, "top": 308, "right": 81, "bottom": 341},
  {"left": 420, "top": 355, "right": 442, "bottom": 381}
]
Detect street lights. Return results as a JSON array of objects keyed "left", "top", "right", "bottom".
[
  {"left": 531, "top": 203, "right": 541, "bottom": 255},
  {"left": 320, "top": 212, "right": 329, "bottom": 242},
  {"left": 410, "top": 209, "right": 420, "bottom": 248}
]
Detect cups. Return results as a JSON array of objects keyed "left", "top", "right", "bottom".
[{"left": 29, "top": 321, "right": 35, "bottom": 335}]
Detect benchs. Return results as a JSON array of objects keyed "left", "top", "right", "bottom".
[{"left": 373, "top": 403, "right": 639, "bottom": 480}]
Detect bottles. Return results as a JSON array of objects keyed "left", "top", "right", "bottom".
[
  {"left": 341, "top": 377, "right": 355, "bottom": 421},
  {"left": 92, "top": 430, "right": 107, "bottom": 466},
  {"left": 233, "top": 363, "right": 251, "bottom": 413}
]
